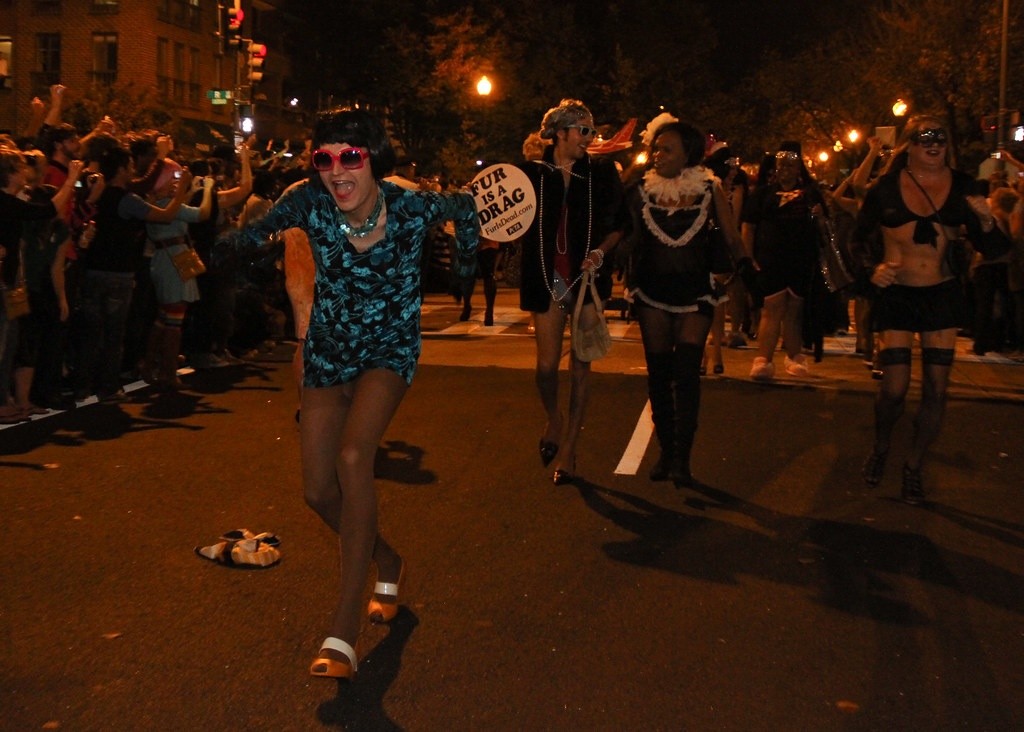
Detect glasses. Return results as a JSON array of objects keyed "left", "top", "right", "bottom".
[
  {"left": 561, "top": 124, "right": 597, "bottom": 138},
  {"left": 311, "top": 147, "right": 371, "bottom": 171}
]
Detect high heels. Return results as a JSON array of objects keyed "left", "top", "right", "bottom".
[
  {"left": 863, "top": 443, "right": 892, "bottom": 488},
  {"left": 311, "top": 636, "right": 359, "bottom": 678},
  {"left": 901, "top": 463, "right": 924, "bottom": 506},
  {"left": 368, "top": 556, "right": 406, "bottom": 623}
]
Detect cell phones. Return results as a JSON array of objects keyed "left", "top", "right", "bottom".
[{"left": 86, "top": 220, "right": 95, "bottom": 231}]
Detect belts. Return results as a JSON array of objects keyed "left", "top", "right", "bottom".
[{"left": 154, "top": 236, "right": 189, "bottom": 251}]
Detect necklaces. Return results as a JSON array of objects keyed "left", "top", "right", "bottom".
[
  {"left": 540, "top": 155, "right": 595, "bottom": 308},
  {"left": 336, "top": 189, "right": 386, "bottom": 239},
  {"left": 903, "top": 162, "right": 948, "bottom": 179}
]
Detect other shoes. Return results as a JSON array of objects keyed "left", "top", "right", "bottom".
[
  {"left": 0, "top": 337, "right": 279, "bottom": 424},
  {"left": 459, "top": 306, "right": 473, "bottom": 323},
  {"left": 715, "top": 366, "right": 725, "bottom": 376},
  {"left": 553, "top": 455, "right": 577, "bottom": 485},
  {"left": 1007, "top": 350, "right": 1024, "bottom": 364},
  {"left": 864, "top": 347, "right": 874, "bottom": 369},
  {"left": 871, "top": 361, "right": 884, "bottom": 379},
  {"left": 727, "top": 330, "right": 746, "bottom": 349},
  {"left": 751, "top": 357, "right": 773, "bottom": 381},
  {"left": 485, "top": 308, "right": 494, "bottom": 326},
  {"left": 784, "top": 353, "right": 810, "bottom": 377},
  {"left": 701, "top": 357, "right": 709, "bottom": 376},
  {"left": 540, "top": 417, "right": 564, "bottom": 467}
]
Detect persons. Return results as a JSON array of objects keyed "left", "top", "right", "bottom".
[
  {"left": 848, "top": 114, "right": 987, "bottom": 503},
  {"left": 519, "top": 123, "right": 1024, "bottom": 375},
  {"left": 268, "top": 109, "right": 482, "bottom": 681},
  {"left": 521, "top": 99, "right": 630, "bottom": 484},
  {"left": 610, "top": 121, "right": 740, "bottom": 492},
  {"left": 0, "top": 85, "right": 500, "bottom": 424}
]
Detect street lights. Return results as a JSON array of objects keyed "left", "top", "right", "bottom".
[
  {"left": 817, "top": 151, "right": 830, "bottom": 183},
  {"left": 474, "top": 74, "right": 494, "bottom": 171},
  {"left": 891, "top": 98, "right": 909, "bottom": 136},
  {"left": 847, "top": 128, "right": 860, "bottom": 172},
  {"left": 832, "top": 139, "right": 845, "bottom": 186}
]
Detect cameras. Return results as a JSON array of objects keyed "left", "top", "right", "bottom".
[
  {"left": 90, "top": 174, "right": 99, "bottom": 184},
  {"left": 200, "top": 179, "right": 206, "bottom": 187},
  {"left": 173, "top": 171, "right": 181, "bottom": 178}
]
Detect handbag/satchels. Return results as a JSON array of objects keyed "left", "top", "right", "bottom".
[
  {"left": 3, "top": 286, "right": 32, "bottom": 320},
  {"left": 947, "top": 244, "right": 970, "bottom": 275},
  {"left": 171, "top": 249, "right": 206, "bottom": 283},
  {"left": 570, "top": 267, "right": 611, "bottom": 361}
]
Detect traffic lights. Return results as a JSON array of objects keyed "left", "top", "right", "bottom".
[
  {"left": 238, "top": 102, "right": 255, "bottom": 136},
  {"left": 246, "top": 41, "right": 267, "bottom": 87},
  {"left": 214, "top": 0, "right": 245, "bottom": 56}
]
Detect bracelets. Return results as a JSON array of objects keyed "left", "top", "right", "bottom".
[
  {"left": 585, "top": 249, "right": 605, "bottom": 269},
  {"left": 64, "top": 184, "right": 74, "bottom": 188}
]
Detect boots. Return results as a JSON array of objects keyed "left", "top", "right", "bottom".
[
  {"left": 644, "top": 352, "right": 675, "bottom": 481},
  {"left": 674, "top": 341, "right": 702, "bottom": 486}
]
